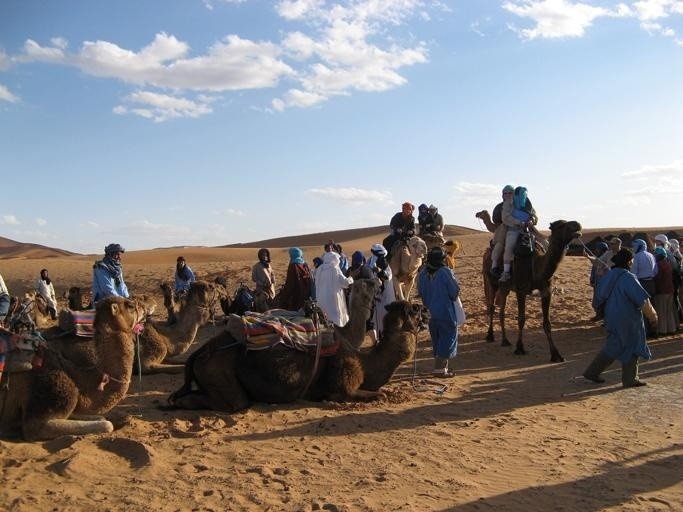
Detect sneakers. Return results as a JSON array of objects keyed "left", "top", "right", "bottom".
[
  {"left": 498, "top": 272, "right": 511, "bottom": 283},
  {"left": 432, "top": 368, "right": 456, "bottom": 378},
  {"left": 489, "top": 267, "right": 502, "bottom": 277}
]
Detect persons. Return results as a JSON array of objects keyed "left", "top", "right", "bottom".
[
  {"left": 496, "top": 186, "right": 549, "bottom": 282},
  {"left": 0, "top": 273, "right": 10, "bottom": 328},
  {"left": 417, "top": 203, "right": 434, "bottom": 236},
  {"left": 34, "top": 268, "right": 57, "bottom": 319},
  {"left": 581, "top": 234, "right": 682, "bottom": 337},
  {"left": 434, "top": 239, "right": 470, "bottom": 331},
  {"left": 582, "top": 249, "right": 658, "bottom": 387},
  {"left": 490, "top": 185, "right": 514, "bottom": 277},
  {"left": 91, "top": 243, "right": 130, "bottom": 306},
  {"left": 427, "top": 203, "right": 444, "bottom": 241},
  {"left": 281, "top": 239, "right": 397, "bottom": 354},
  {"left": 173, "top": 255, "right": 195, "bottom": 301},
  {"left": 251, "top": 248, "right": 275, "bottom": 300},
  {"left": 416, "top": 247, "right": 460, "bottom": 378},
  {"left": 388, "top": 202, "right": 415, "bottom": 240}
]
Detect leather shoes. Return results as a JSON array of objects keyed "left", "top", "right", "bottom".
[
  {"left": 583, "top": 371, "right": 605, "bottom": 383},
  {"left": 632, "top": 380, "right": 647, "bottom": 386}
]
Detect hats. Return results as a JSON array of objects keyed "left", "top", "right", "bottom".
[{"left": 607, "top": 237, "right": 622, "bottom": 245}]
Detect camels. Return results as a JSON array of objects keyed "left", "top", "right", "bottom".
[
  {"left": 389, "top": 234, "right": 459, "bottom": 301},
  {"left": 480, "top": 219, "right": 583, "bottom": 364},
  {"left": 0, "top": 277, "right": 382, "bottom": 375},
  {"left": 1, "top": 280, "right": 432, "bottom": 444},
  {"left": 0, "top": 291, "right": 158, "bottom": 442},
  {"left": 167, "top": 300, "right": 431, "bottom": 411},
  {"left": 475, "top": 209, "right": 498, "bottom": 232}
]
